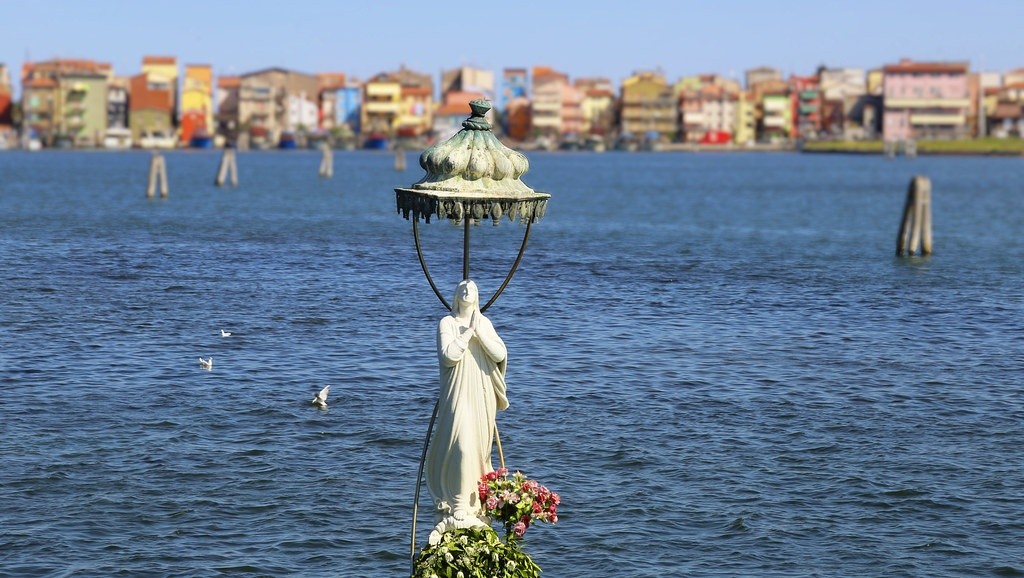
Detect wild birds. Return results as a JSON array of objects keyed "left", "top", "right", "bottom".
[
  {"left": 220, "top": 329, "right": 231, "bottom": 337},
  {"left": 311, "top": 385, "right": 331, "bottom": 405},
  {"left": 199, "top": 357, "right": 213, "bottom": 366}
]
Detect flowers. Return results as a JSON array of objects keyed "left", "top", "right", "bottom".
[{"left": 410, "top": 468, "right": 560, "bottom": 578}]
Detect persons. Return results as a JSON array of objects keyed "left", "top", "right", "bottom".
[{"left": 425, "top": 279, "right": 510, "bottom": 546}]
